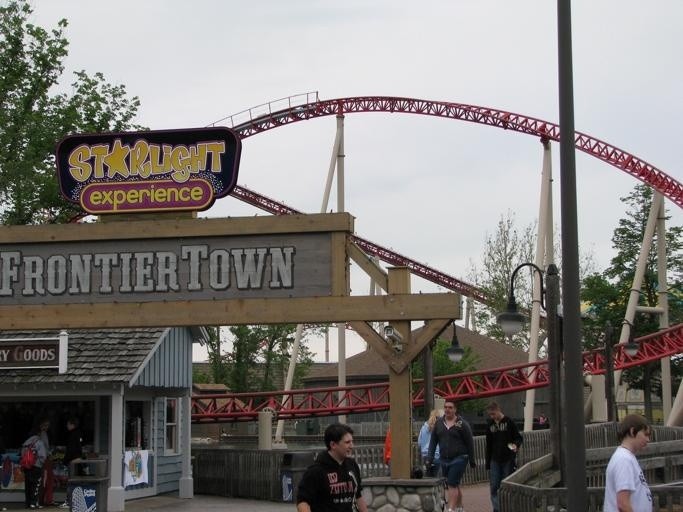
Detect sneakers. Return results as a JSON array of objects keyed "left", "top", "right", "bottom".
[
  {"left": 25, "top": 502, "right": 46, "bottom": 509},
  {"left": 57, "top": 500, "right": 71, "bottom": 509}
]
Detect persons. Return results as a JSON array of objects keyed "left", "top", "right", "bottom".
[
  {"left": 540, "top": 414, "right": 551, "bottom": 429},
  {"left": 604, "top": 414, "right": 653, "bottom": 512},
  {"left": 418, "top": 400, "right": 477, "bottom": 512},
  {"left": 484, "top": 401, "right": 523, "bottom": 512},
  {"left": 297, "top": 424, "right": 368, "bottom": 512},
  {"left": 21, "top": 419, "right": 83, "bottom": 509}
]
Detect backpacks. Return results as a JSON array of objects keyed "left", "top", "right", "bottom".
[{"left": 20, "top": 440, "right": 40, "bottom": 469}]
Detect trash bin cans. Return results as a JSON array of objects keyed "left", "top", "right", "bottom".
[
  {"left": 281, "top": 452, "right": 314, "bottom": 502},
  {"left": 67, "top": 459, "right": 107, "bottom": 512}
]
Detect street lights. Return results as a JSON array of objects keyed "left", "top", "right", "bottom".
[
  {"left": 496, "top": 263, "right": 560, "bottom": 467},
  {"left": 424, "top": 320, "right": 464, "bottom": 421},
  {"left": 605, "top": 318, "right": 639, "bottom": 422}
]
